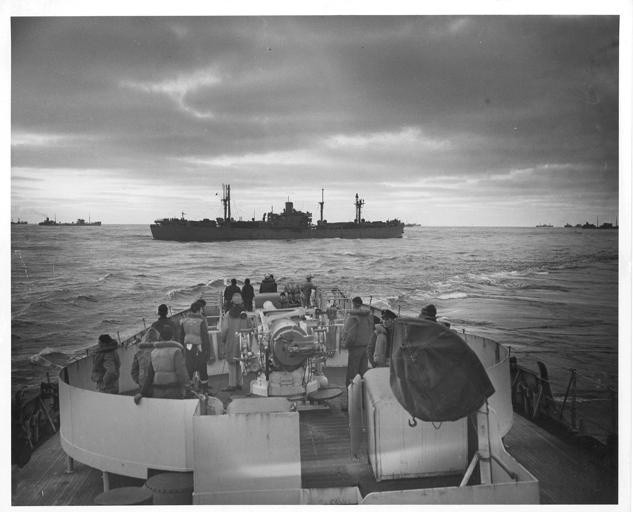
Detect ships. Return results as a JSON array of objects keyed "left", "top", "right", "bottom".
[
  {"left": 39, "top": 218, "right": 102, "bottom": 226},
  {"left": 12, "top": 282, "right": 618, "bottom": 503},
  {"left": 150, "top": 184, "right": 404, "bottom": 242}
]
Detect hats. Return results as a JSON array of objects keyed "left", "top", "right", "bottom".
[
  {"left": 381, "top": 309, "right": 397, "bottom": 320},
  {"left": 421, "top": 304, "right": 437, "bottom": 314},
  {"left": 230, "top": 292, "right": 244, "bottom": 305}
]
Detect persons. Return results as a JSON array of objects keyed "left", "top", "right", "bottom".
[
  {"left": 178, "top": 304, "right": 214, "bottom": 396},
  {"left": 194, "top": 299, "right": 213, "bottom": 388},
  {"left": 130, "top": 328, "right": 161, "bottom": 390},
  {"left": 133, "top": 324, "right": 189, "bottom": 405},
  {"left": 150, "top": 304, "right": 176, "bottom": 340},
  {"left": 340, "top": 297, "right": 370, "bottom": 411},
  {"left": 241, "top": 278, "right": 254, "bottom": 311},
  {"left": 269, "top": 274, "right": 277, "bottom": 290},
  {"left": 417, "top": 303, "right": 437, "bottom": 323},
  {"left": 300, "top": 272, "right": 316, "bottom": 306},
  {"left": 223, "top": 278, "right": 240, "bottom": 311},
  {"left": 366, "top": 309, "right": 398, "bottom": 366},
  {"left": 90, "top": 334, "right": 121, "bottom": 394},
  {"left": 278, "top": 292, "right": 289, "bottom": 307},
  {"left": 218, "top": 292, "right": 247, "bottom": 392},
  {"left": 259, "top": 272, "right": 276, "bottom": 292}
]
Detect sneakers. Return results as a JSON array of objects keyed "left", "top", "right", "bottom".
[{"left": 198, "top": 381, "right": 243, "bottom": 392}]
